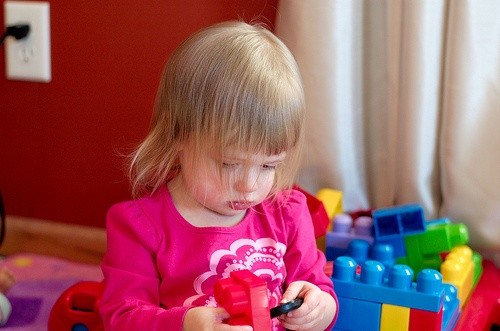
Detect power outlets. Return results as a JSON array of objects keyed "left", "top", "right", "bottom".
[{"left": 4, "top": 2, "right": 50, "bottom": 81}]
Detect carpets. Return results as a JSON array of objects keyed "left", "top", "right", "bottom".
[{"left": 0, "top": 252, "right": 105, "bottom": 331}]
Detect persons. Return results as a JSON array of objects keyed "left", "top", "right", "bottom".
[{"left": 100, "top": 20, "right": 341, "bottom": 331}]
